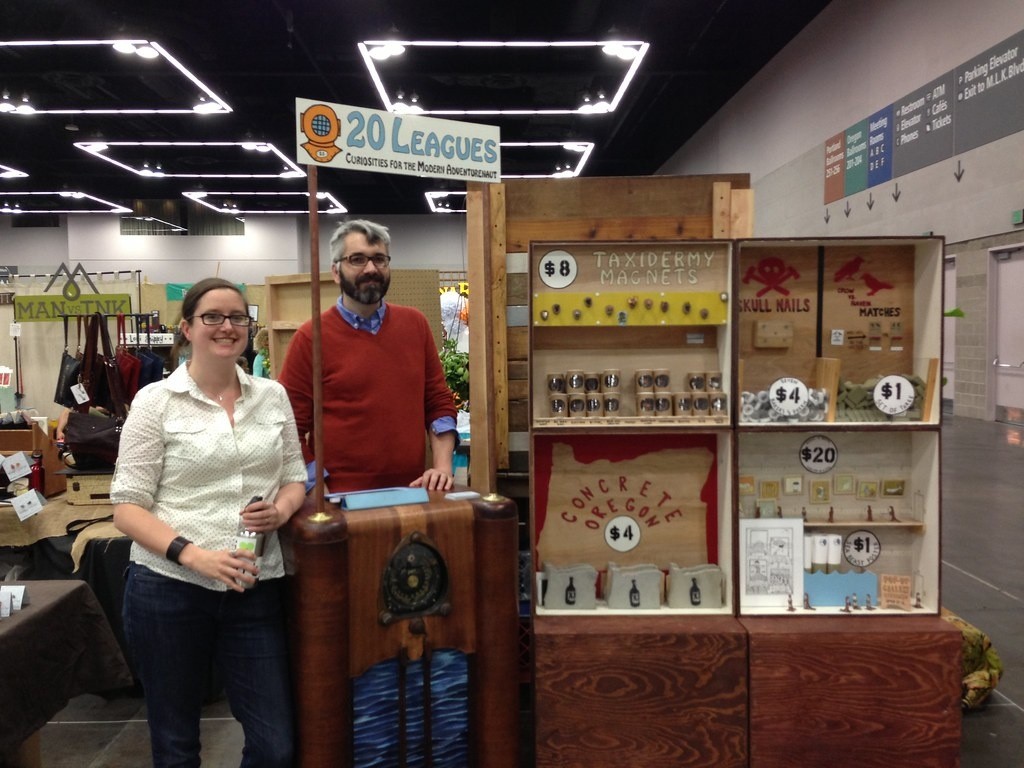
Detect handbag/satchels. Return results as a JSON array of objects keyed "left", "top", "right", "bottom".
[{"left": 65, "top": 413, "right": 128, "bottom": 464}]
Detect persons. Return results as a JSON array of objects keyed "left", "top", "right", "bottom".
[
  {"left": 275, "top": 220, "right": 461, "bottom": 492},
  {"left": 110, "top": 278, "right": 310, "bottom": 768},
  {"left": 56, "top": 327, "right": 272, "bottom": 442}
]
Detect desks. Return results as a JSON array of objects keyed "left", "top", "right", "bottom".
[{"left": 0, "top": 580, "right": 87, "bottom": 768}]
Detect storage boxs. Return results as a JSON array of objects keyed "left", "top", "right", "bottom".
[{"left": 66, "top": 475, "right": 114, "bottom": 505}]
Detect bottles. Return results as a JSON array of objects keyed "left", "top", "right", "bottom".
[
  {"left": 232, "top": 496, "right": 266, "bottom": 589},
  {"left": 28, "top": 449, "right": 45, "bottom": 495}
]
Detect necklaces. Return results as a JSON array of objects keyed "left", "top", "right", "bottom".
[{"left": 195, "top": 381, "right": 234, "bottom": 401}]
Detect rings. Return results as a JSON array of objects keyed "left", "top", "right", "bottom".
[{"left": 231, "top": 549, "right": 238, "bottom": 556}]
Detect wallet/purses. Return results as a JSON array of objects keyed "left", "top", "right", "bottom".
[{"left": 54, "top": 311, "right": 165, "bottom": 408}]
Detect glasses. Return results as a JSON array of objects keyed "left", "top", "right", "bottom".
[
  {"left": 335, "top": 252, "right": 391, "bottom": 268},
  {"left": 192, "top": 313, "right": 253, "bottom": 327}
]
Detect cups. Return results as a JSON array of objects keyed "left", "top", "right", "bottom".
[{"left": 546, "top": 368, "right": 727, "bottom": 417}]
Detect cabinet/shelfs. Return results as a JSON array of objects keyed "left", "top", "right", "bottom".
[{"left": 528, "top": 235, "right": 963, "bottom": 768}]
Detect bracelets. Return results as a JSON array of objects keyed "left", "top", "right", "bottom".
[{"left": 166, "top": 535, "right": 192, "bottom": 566}]
